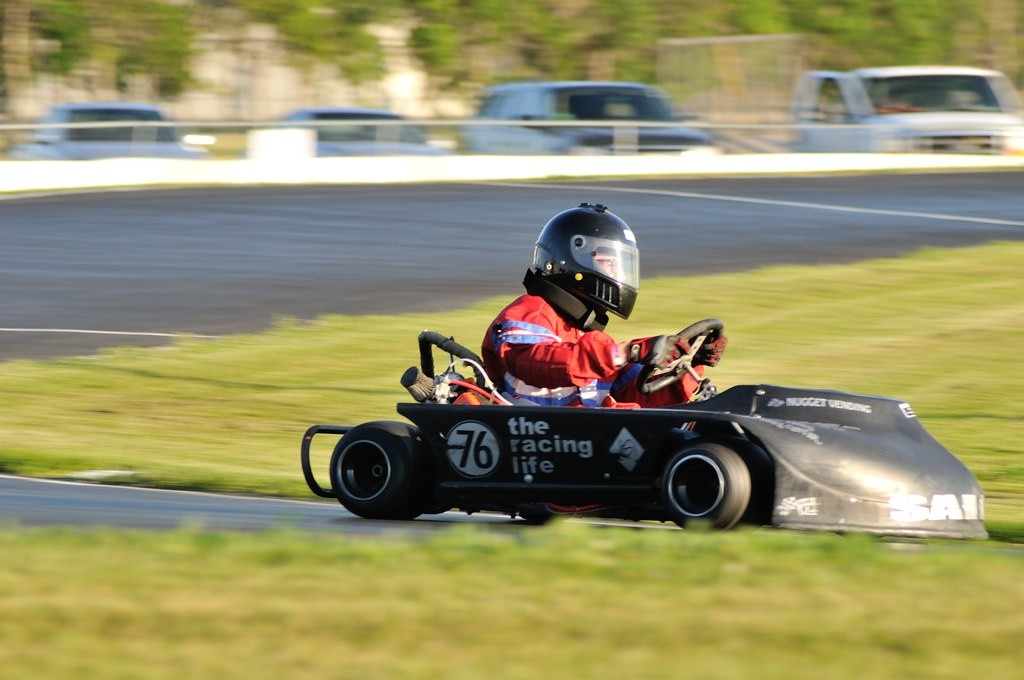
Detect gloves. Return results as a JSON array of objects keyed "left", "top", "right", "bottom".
[
  {"left": 627, "top": 334, "right": 692, "bottom": 370},
  {"left": 694, "top": 334, "right": 728, "bottom": 367}
]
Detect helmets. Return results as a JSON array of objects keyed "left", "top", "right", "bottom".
[{"left": 532, "top": 202, "right": 641, "bottom": 320}]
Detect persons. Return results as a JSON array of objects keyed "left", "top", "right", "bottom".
[{"left": 481, "top": 203, "right": 729, "bottom": 409}]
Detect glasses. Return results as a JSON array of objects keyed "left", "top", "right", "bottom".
[{"left": 596, "top": 258, "right": 624, "bottom": 275}]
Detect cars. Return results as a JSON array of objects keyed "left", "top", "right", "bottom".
[
  {"left": 780, "top": 66, "right": 1024, "bottom": 155},
  {"left": 5, "top": 101, "right": 209, "bottom": 160},
  {"left": 458, "top": 79, "right": 717, "bottom": 156},
  {"left": 241, "top": 105, "right": 453, "bottom": 157}
]
